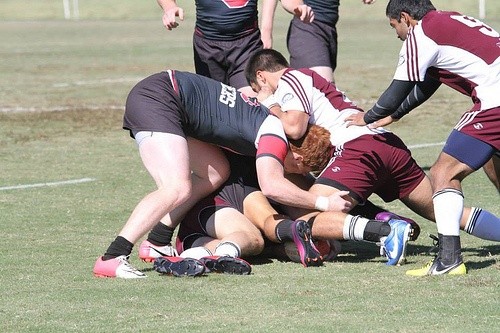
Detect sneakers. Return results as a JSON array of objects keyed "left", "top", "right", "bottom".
[
  {"left": 139, "top": 240, "right": 179, "bottom": 263},
  {"left": 376, "top": 219, "right": 415, "bottom": 266},
  {"left": 154, "top": 256, "right": 205, "bottom": 276},
  {"left": 93, "top": 255, "right": 147, "bottom": 278},
  {"left": 375, "top": 213, "right": 420, "bottom": 240},
  {"left": 406, "top": 254, "right": 466, "bottom": 276},
  {"left": 292, "top": 219, "right": 324, "bottom": 267},
  {"left": 200, "top": 255, "right": 252, "bottom": 275}
]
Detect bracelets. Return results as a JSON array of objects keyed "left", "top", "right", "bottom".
[{"left": 314, "top": 196, "right": 330, "bottom": 212}]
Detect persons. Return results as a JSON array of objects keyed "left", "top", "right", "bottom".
[
  {"left": 280, "top": 0, "right": 341, "bottom": 91},
  {"left": 156, "top": 0, "right": 278, "bottom": 96},
  {"left": 92, "top": 48, "right": 500, "bottom": 280},
  {"left": 342, "top": 0, "right": 500, "bottom": 278}
]
